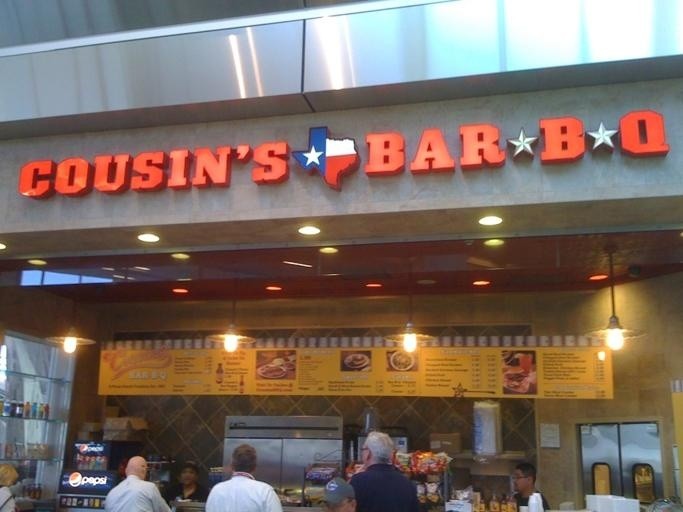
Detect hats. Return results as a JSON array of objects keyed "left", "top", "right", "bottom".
[
  {"left": 317, "top": 478, "right": 355, "bottom": 505},
  {"left": 181, "top": 460, "right": 198, "bottom": 470}
]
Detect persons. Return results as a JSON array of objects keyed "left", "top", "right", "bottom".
[
  {"left": 323, "top": 474, "right": 357, "bottom": 512},
  {"left": 349, "top": 430, "right": 419, "bottom": 512},
  {"left": 0, "top": 484, "right": 20, "bottom": 511},
  {"left": 510, "top": 461, "right": 550, "bottom": 512},
  {"left": 169, "top": 459, "right": 209, "bottom": 503},
  {"left": 0, "top": 464, "right": 18, "bottom": 486},
  {"left": 204, "top": 443, "right": 283, "bottom": 512},
  {"left": 104, "top": 454, "right": 172, "bottom": 511}
]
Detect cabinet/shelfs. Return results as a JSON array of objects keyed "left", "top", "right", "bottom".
[{"left": 0, "top": 370, "right": 66, "bottom": 504}]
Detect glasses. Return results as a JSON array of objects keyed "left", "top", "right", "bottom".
[{"left": 512, "top": 476, "right": 528, "bottom": 480}]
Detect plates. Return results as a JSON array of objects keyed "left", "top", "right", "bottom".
[
  {"left": 343, "top": 354, "right": 371, "bottom": 369},
  {"left": 257, "top": 364, "right": 287, "bottom": 379},
  {"left": 504, "top": 377, "right": 529, "bottom": 393},
  {"left": 390, "top": 351, "right": 415, "bottom": 370}
]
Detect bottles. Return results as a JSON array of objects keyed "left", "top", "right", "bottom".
[
  {"left": 0, "top": 399, "right": 50, "bottom": 419},
  {"left": 528, "top": 493, "right": 545, "bottom": 512},
  {"left": 22, "top": 483, "right": 42, "bottom": 500},
  {"left": 0, "top": 440, "right": 51, "bottom": 459}
]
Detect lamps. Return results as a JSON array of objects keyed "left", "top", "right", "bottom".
[
  {"left": 209, "top": 254, "right": 255, "bottom": 353},
  {"left": 43, "top": 254, "right": 97, "bottom": 354},
  {"left": 588, "top": 242, "right": 646, "bottom": 346},
  {"left": 387, "top": 251, "right": 433, "bottom": 350}
]
